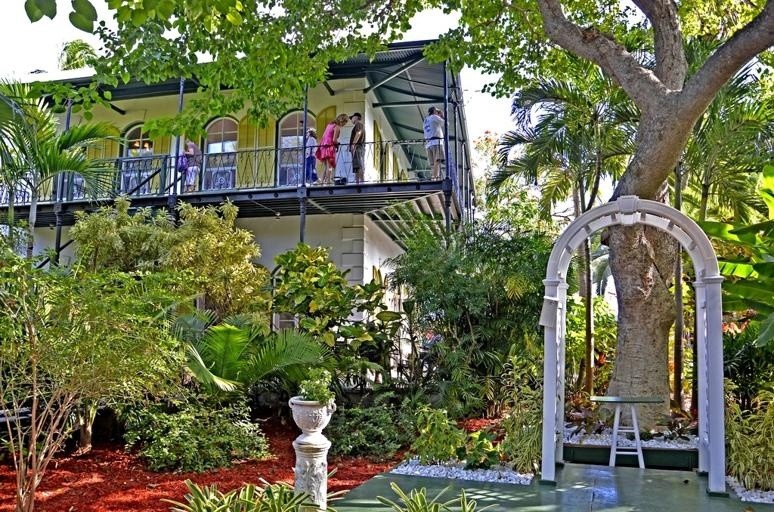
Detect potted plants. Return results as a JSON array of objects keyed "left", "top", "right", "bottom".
[{"left": 288, "top": 366, "right": 337, "bottom": 445}]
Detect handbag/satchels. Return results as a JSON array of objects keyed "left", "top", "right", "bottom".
[{"left": 178, "top": 151, "right": 188, "bottom": 172}]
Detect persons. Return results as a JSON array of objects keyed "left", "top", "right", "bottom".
[
  {"left": 315, "top": 114, "right": 349, "bottom": 183},
  {"left": 423, "top": 107, "right": 451, "bottom": 179},
  {"left": 184, "top": 137, "right": 203, "bottom": 193},
  {"left": 304, "top": 128, "right": 318, "bottom": 184},
  {"left": 350, "top": 112, "right": 366, "bottom": 183}
]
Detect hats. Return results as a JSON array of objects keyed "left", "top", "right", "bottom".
[
  {"left": 349, "top": 112, "right": 362, "bottom": 119},
  {"left": 306, "top": 128, "right": 316, "bottom": 132},
  {"left": 428, "top": 106, "right": 441, "bottom": 111}
]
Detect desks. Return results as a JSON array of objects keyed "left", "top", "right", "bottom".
[{"left": 589, "top": 395, "right": 666, "bottom": 469}]
